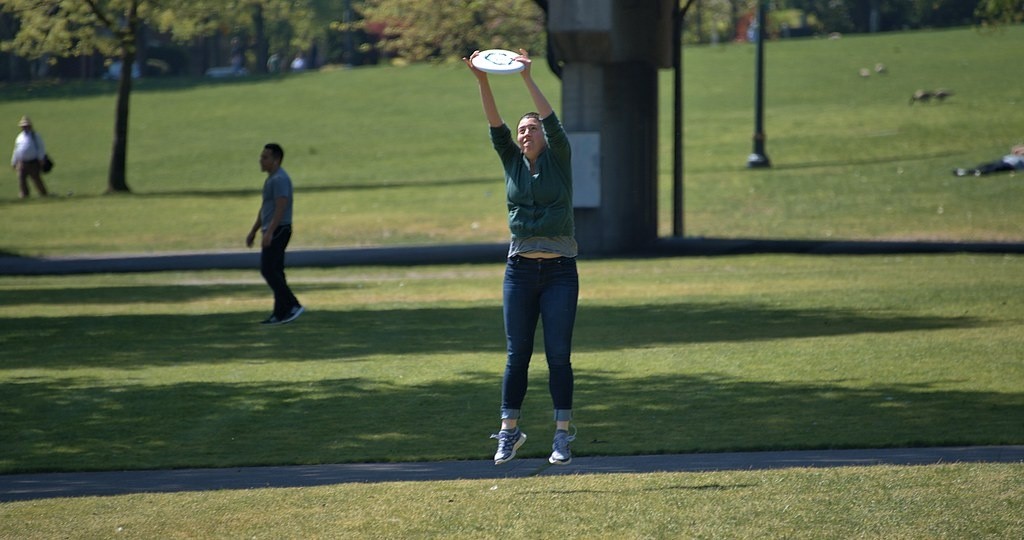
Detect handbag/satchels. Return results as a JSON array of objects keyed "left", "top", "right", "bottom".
[{"left": 41, "top": 154, "right": 53, "bottom": 173}]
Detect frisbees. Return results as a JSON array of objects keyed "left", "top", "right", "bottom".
[{"left": 471, "top": 48, "right": 526, "bottom": 75}]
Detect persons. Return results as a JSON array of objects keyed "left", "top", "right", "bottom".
[
  {"left": 10, "top": 116, "right": 47, "bottom": 198},
  {"left": 951, "top": 145, "right": 1024, "bottom": 176},
  {"left": 463, "top": 46, "right": 579, "bottom": 464},
  {"left": 246, "top": 142, "right": 305, "bottom": 326}
]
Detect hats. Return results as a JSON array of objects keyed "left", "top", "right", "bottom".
[{"left": 18, "top": 116, "right": 31, "bottom": 127}]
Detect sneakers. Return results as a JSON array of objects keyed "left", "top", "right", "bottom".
[
  {"left": 259, "top": 314, "right": 280, "bottom": 326},
  {"left": 281, "top": 305, "right": 304, "bottom": 324},
  {"left": 489, "top": 426, "right": 526, "bottom": 465},
  {"left": 549, "top": 428, "right": 575, "bottom": 464}
]
